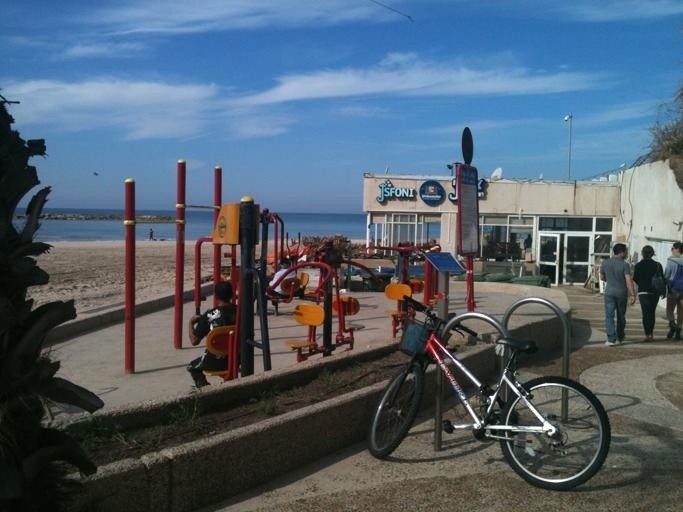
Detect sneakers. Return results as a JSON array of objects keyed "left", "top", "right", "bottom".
[
  {"left": 667, "top": 327, "right": 675, "bottom": 338},
  {"left": 605, "top": 340, "right": 616, "bottom": 346}
]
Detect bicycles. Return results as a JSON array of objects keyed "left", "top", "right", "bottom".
[{"left": 369, "top": 293, "right": 612, "bottom": 492}]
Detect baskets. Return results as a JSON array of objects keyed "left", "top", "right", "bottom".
[{"left": 398, "top": 314, "right": 452, "bottom": 364}]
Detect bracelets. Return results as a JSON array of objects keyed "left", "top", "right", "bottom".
[{"left": 630, "top": 293, "right": 635, "bottom": 296}]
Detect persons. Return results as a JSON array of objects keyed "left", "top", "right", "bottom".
[
  {"left": 268, "top": 258, "right": 297, "bottom": 298},
  {"left": 148, "top": 228, "right": 155, "bottom": 240},
  {"left": 663, "top": 242, "right": 683, "bottom": 342},
  {"left": 598, "top": 242, "right": 638, "bottom": 347},
  {"left": 186, "top": 280, "right": 241, "bottom": 392},
  {"left": 317, "top": 236, "right": 341, "bottom": 297},
  {"left": 523, "top": 233, "right": 532, "bottom": 248},
  {"left": 632, "top": 245, "right": 666, "bottom": 340}
]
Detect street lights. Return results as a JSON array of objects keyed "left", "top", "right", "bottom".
[{"left": 565, "top": 113, "right": 574, "bottom": 183}]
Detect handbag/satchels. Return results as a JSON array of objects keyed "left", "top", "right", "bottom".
[{"left": 650, "top": 262, "right": 665, "bottom": 292}]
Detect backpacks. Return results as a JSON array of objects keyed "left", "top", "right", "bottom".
[{"left": 668, "top": 258, "right": 683, "bottom": 290}]
